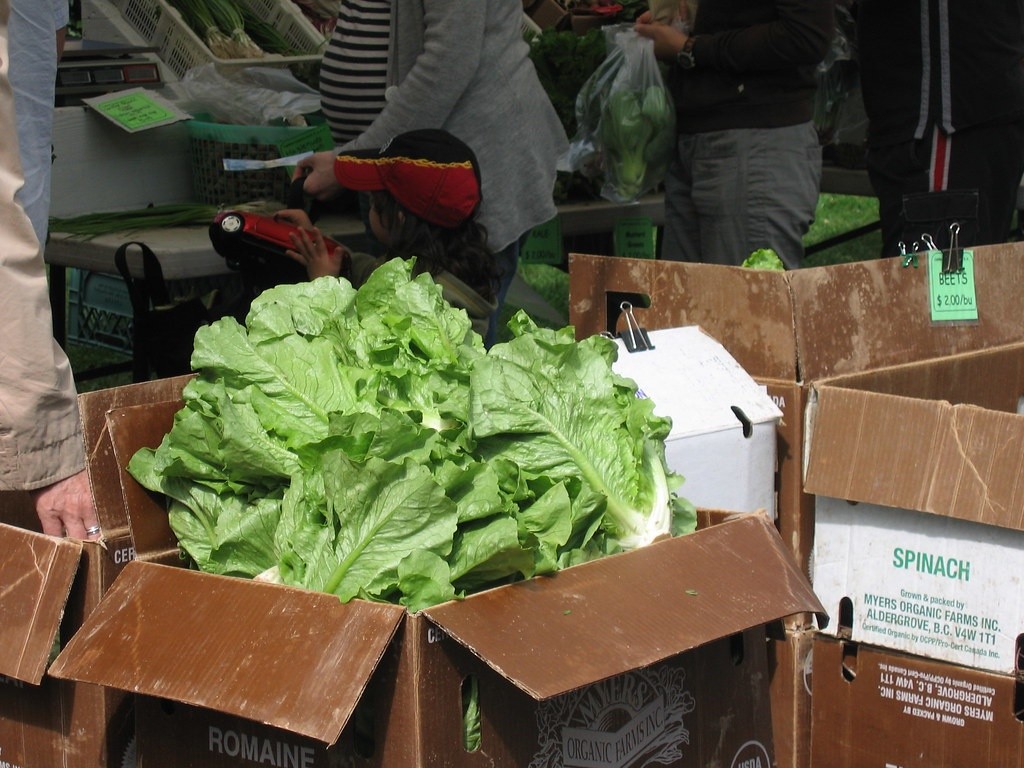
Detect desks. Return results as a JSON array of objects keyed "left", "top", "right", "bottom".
[{"left": 46, "top": 190, "right": 667, "bottom": 383}]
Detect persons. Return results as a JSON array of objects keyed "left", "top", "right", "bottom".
[
  {"left": 854, "top": 0, "right": 1024, "bottom": 257},
  {"left": 274, "top": 127, "right": 507, "bottom": 353},
  {"left": 290, "top": 0, "right": 571, "bottom": 350},
  {"left": 633, "top": 0, "right": 834, "bottom": 273},
  {"left": 1, "top": 0, "right": 102, "bottom": 545}
]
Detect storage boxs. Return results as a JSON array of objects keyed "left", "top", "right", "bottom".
[
  {"left": 91, "top": 0, "right": 328, "bottom": 90},
  {"left": 809, "top": 633, "right": 1024, "bottom": 768},
  {"left": 569, "top": 241, "right": 1024, "bottom": 768},
  {"left": 48, "top": 0, "right": 209, "bottom": 222},
  {"left": 45, "top": 397, "right": 828, "bottom": 768},
  {"left": 67, "top": 266, "right": 237, "bottom": 359},
  {"left": 804, "top": 341, "right": 1024, "bottom": 676},
  {"left": 604, "top": 323, "right": 779, "bottom": 528},
  {"left": 0, "top": 371, "right": 199, "bottom": 768},
  {"left": 523, "top": 0, "right": 622, "bottom": 33}
]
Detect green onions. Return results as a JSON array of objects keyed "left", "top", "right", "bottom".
[
  {"left": 166, "top": 0, "right": 322, "bottom": 61},
  {"left": 45, "top": 202, "right": 268, "bottom": 234}
]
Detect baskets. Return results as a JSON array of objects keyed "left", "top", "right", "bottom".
[
  {"left": 182, "top": 109, "right": 325, "bottom": 207},
  {"left": 111, "top": 0, "right": 327, "bottom": 82}
]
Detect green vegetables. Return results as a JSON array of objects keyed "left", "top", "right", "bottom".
[{"left": 523, "top": 27, "right": 854, "bottom": 272}]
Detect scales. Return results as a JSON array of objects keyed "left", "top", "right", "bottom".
[{"left": 55, "top": 1, "right": 165, "bottom": 110}]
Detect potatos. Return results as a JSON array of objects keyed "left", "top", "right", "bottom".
[{"left": 187, "top": 115, "right": 308, "bottom": 203}]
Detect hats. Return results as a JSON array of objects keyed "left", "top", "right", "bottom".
[{"left": 334, "top": 127, "right": 481, "bottom": 229}]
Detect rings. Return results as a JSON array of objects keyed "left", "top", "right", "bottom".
[{"left": 86, "top": 524, "right": 100, "bottom": 535}]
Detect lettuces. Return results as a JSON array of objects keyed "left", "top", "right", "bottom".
[{"left": 126, "top": 257, "right": 697, "bottom": 750}]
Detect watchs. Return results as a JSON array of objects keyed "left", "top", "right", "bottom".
[{"left": 676, "top": 36, "right": 695, "bottom": 69}]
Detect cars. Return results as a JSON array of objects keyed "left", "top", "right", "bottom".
[{"left": 209, "top": 210, "right": 352, "bottom": 270}]
